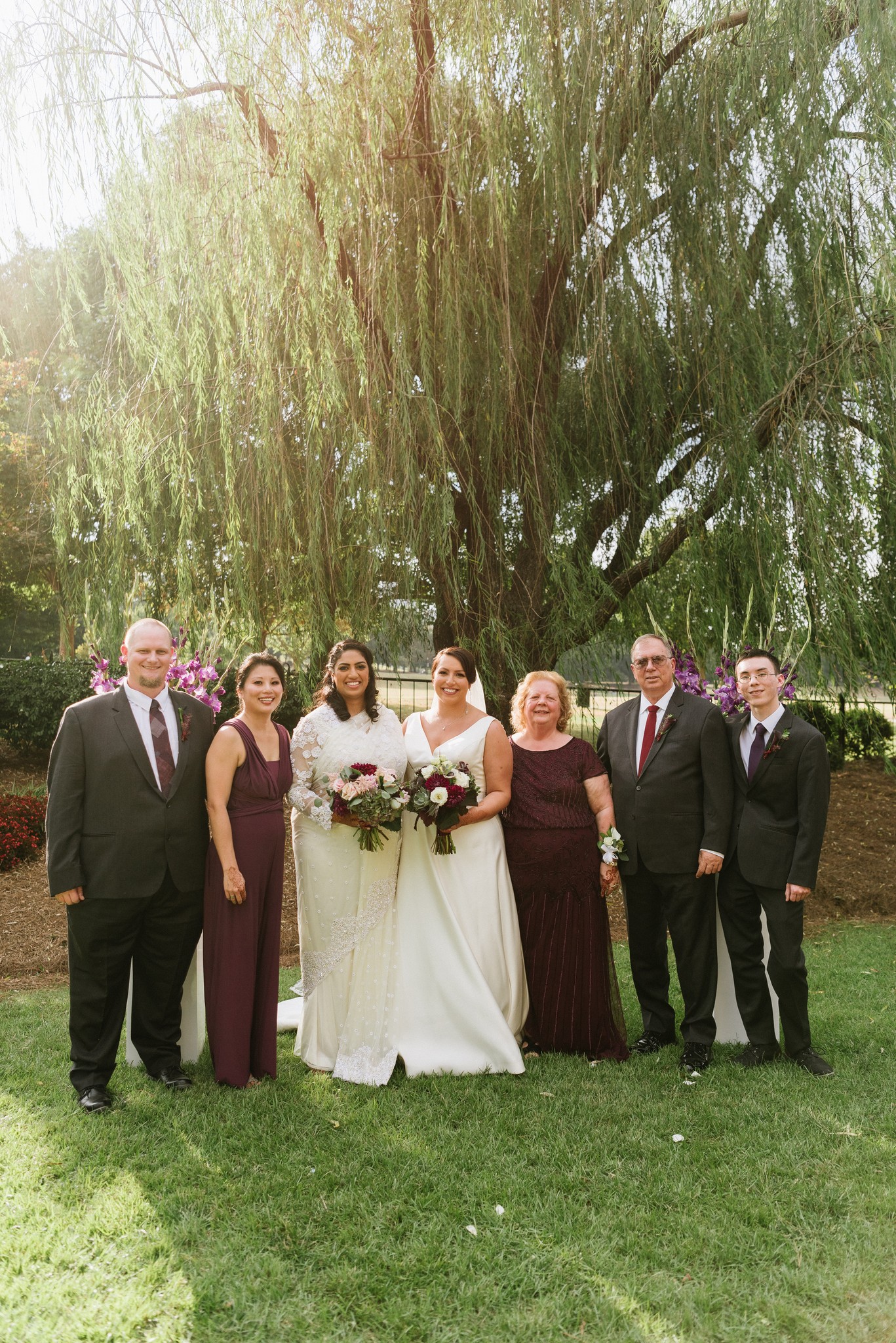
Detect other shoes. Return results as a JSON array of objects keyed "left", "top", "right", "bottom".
[{"left": 522, "top": 1035, "right": 544, "bottom": 1061}]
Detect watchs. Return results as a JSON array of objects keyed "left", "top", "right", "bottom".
[{"left": 601, "top": 859, "right": 618, "bottom": 867}]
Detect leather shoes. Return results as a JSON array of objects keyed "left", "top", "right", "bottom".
[
  {"left": 678, "top": 1042, "right": 713, "bottom": 1074},
  {"left": 77, "top": 1085, "right": 112, "bottom": 1115},
  {"left": 628, "top": 1030, "right": 677, "bottom": 1055},
  {"left": 144, "top": 1066, "right": 192, "bottom": 1092}
]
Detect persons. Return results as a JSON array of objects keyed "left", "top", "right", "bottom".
[
  {"left": 501, "top": 669, "right": 632, "bottom": 1061},
  {"left": 718, "top": 648, "right": 836, "bottom": 1076},
  {"left": 287, "top": 639, "right": 409, "bottom": 1088},
  {"left": 45, "top": 617, "right": 216, "bottom": 1110},
  {"left": 598, "top": 633, "right": 734, "bottom": 1070},
  {"left": 202, "top": 652, "right": 295, "bottom": 1084},
  {"left": 399, "top": 645, "right": 531, "bottom": 1078}
]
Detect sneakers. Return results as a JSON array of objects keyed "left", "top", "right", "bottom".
[
  {"left": 784, "top": 1041, "right": 835, "bottom": 1079},
  {"left": 723, "top": 1040, "right": 782, "bottom": 1071}
]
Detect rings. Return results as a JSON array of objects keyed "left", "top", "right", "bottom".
[
  {"left": 231, "top": 896, "right": 235, "bottom": 899},
  {"left": 617, "top": 884, "right": 618, "bottom": 886},
  {"left": 357, "top": 823, "right": 361, "bottom": 828},
  {"left": 609, "top": 883, "right": 618, "bottom": 888}
]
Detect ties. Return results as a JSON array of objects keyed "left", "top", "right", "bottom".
[
  {"left": 149, "top": 699, "right": 175, "bottom": 800},
  {"left": 638, "top": 705, "right": 660, "bottom": 779},
  {"left": 748, "top": 723, "right": 768, "bottom": 788}
]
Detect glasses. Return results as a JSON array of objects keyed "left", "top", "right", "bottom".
[{"left": 632, "top": 655, "right": 671, "bottom": 670}]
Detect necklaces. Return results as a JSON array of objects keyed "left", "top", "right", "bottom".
[{"left": 436, "top": 703, "right": 468, "bottom": 730}]
